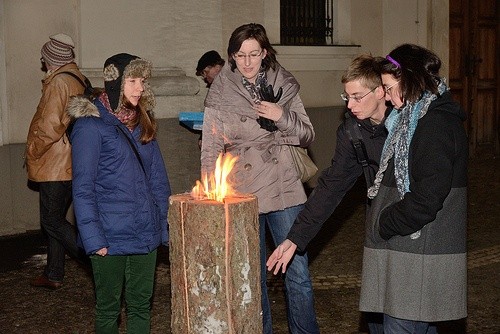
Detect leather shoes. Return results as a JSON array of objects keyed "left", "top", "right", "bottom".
[{"left": 31, "top": 275, "right": 62, "bottom": 290}]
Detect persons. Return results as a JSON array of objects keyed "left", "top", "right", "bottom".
[
  {"left": 67, "top": 53, "right": 171, "bottom": 334},
  {"left": 196, "top": 50, "right": 226, "bottom": 149},
  {"left": 25, "top": 33, "right": 92, "bottom": 289},
  {"left": 266, "top": 51, "right": 394, "bottom": 334},
  {"left": 358, "top": 44, "right": 469, "bottom": 334},
  {"left": 201, "top": 23, "right": 319, "bottom": 334}
]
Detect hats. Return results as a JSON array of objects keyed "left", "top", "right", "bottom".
[
  {"left": 196, "top": 51, "right": 223, "bottom": 74},
  {"left": 103, "top": 53, "right": 155, "bottom": 113},
  {"left": 41, "top": 33, "right": 75, "bottom": 67}
]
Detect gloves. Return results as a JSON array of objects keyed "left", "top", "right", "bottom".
[{"left": 260, "top": 85, "right": 282, "bottom": 132}]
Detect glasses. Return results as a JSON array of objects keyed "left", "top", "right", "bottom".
[
  {"left": 341, "top": 86, "right": 378, "bottom": 103},
  {"left": 383, "top": 80, "right": 399, "bottom": 94},
  {"left": 234, "top": 50, "right": 263, "bottom": 60}
]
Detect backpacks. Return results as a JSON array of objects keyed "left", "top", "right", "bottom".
[{"left": 51, "top": 71, "right": 103, "bottom": 145}]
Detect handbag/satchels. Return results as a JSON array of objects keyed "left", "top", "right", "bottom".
[{"left": 292, "top": 145, "right": 318, "bottom": 184}]
